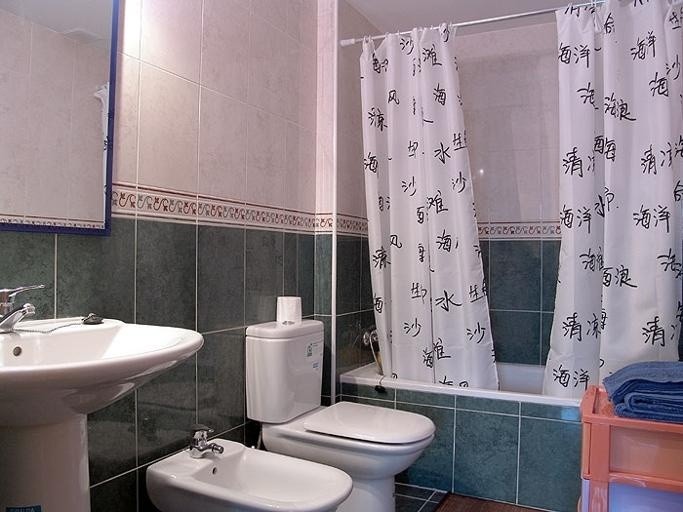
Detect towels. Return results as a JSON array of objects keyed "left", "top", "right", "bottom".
[{"left": 602, "top": 359, "right": 681, "bottom": 425}]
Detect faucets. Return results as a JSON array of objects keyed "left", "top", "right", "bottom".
[
  {"left": 128, "top": 412, "right": 158, "bottom": 439},
  {"left": 0, "top": 283, "right": 47, "bottom": 333},
  {"left": 189, "top": 422, "right": 226, "bottom": 459},
  {"left": 360, "top": 330, "right": 378, "bottom": 346}
]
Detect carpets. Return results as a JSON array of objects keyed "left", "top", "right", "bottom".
[{"left": 428, "top": 491, "right": 544, "bottom": 512}]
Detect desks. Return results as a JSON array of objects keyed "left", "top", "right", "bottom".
[{"left": 580, "top": 381, "right": 683, "bottom": 511}]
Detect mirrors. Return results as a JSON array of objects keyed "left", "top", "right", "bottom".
[{"left": 0, "top": 1, "right": 119, "bottom": 237}]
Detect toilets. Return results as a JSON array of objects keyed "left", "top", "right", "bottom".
[{"left": 243, "top": 312, "right": 436, "bottom": 512}]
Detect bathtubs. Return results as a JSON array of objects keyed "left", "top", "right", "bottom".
[{"left": 340, "top": 360, "right": 583, "bottom": 413}]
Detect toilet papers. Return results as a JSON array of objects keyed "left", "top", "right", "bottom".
[{"left": 277, "top": 295, "right": 301, "bottom": 323}]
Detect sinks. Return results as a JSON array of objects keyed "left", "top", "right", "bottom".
[
  {"left": 0, "top": 316, "right": 204, "bottom": 373},
  {"left": 147, "top": 442, "right": 354, "bottom": 511},
  {"left": 84, "top": 420, "right": 188, "bottom": 468}
]
